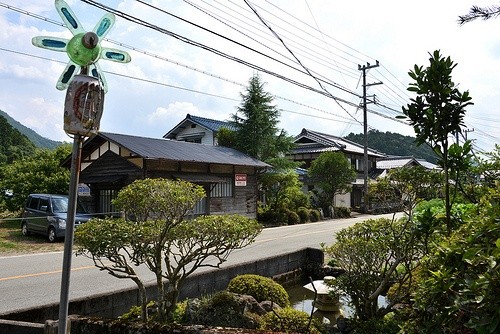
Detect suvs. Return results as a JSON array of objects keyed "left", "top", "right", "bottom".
[{"left": 20, "top": 193, "right": 96, "bottom": 243}]
